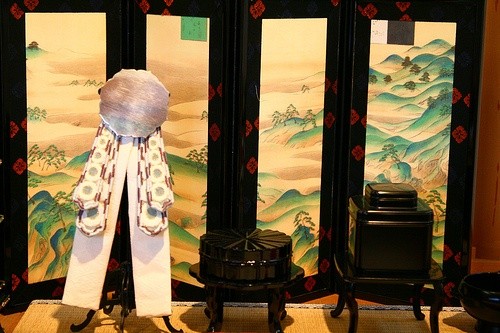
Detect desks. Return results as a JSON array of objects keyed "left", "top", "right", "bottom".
[
  {"left": 330, "top": 249, "right": 444, "bottom": 332},
  {"left": 189, "top": 261, "right": 305, "bottom": 333}
]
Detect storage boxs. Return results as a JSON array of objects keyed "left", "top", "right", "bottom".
[
  {"left": 365, "top": 182, "right": 418, "bottom": 208},
  {"left": 199, "top": 228, "right": 292, "bottom": 280},
  {"left": 348, "top": 195, "right": 434, "bottom": 271}
]
